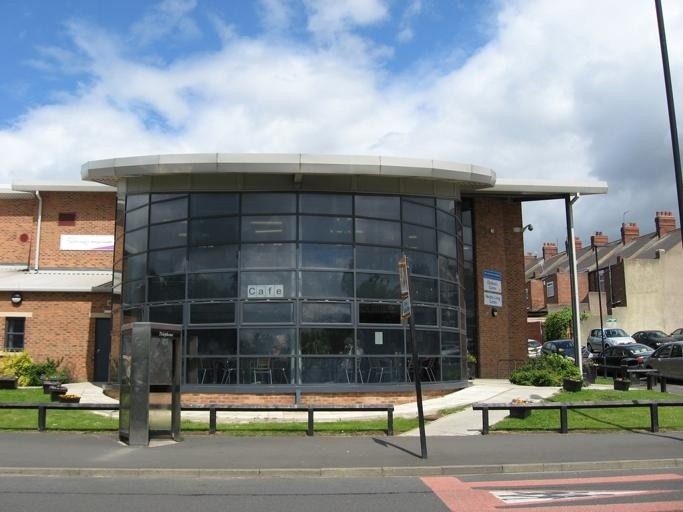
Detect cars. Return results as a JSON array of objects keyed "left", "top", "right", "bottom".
[
  {"left": 527, "top": 337, "right": 683, "bottom": 381},
  {"left": 667, "top": 327, "right": 683, "bottom": 340},
  {"left": 629, "top": 329, "right": 675, "bottom": 350},
  {"left": 585, "top": 327, "right": 635, "bottom": 350}
]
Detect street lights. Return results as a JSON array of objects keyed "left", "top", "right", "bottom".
[{"left": 590, "top": 244, "right": 609, "bottom": 380}]
